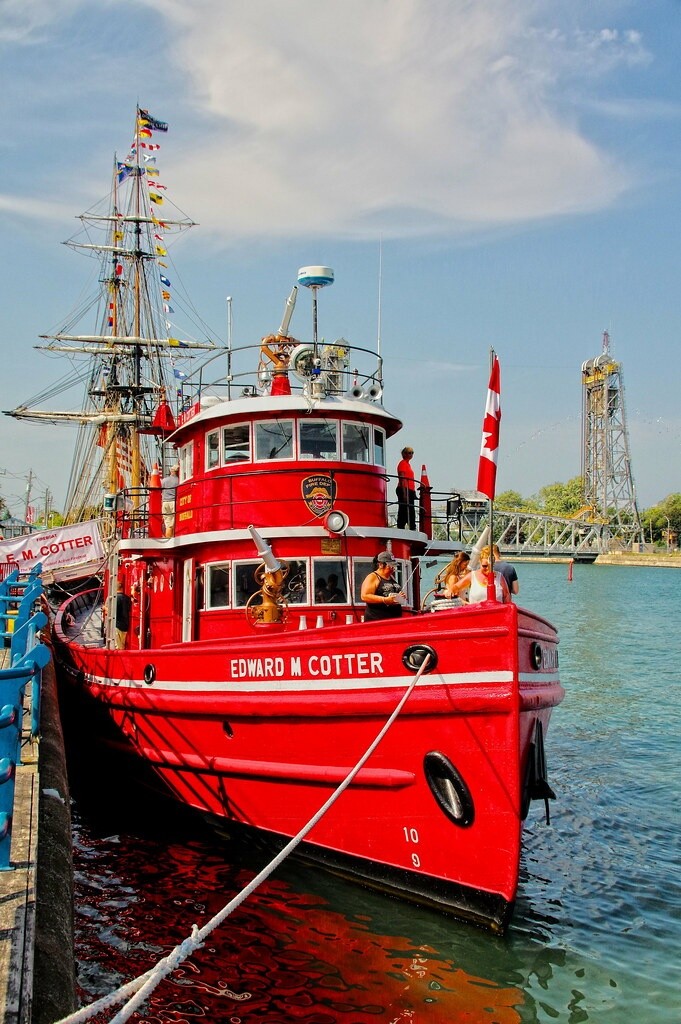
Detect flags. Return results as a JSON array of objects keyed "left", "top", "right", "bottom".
[
  {"left": 102, "top": 101, "right": 199, "bottom": 399},
  {"left": 476, "top": 355, "right": 501, "bottom": 502},
  {"left": 26, "top": 505, "right": 36, "bottom": 525},
  {"left": 97, "top": 386, "right": 151, "bottom": 538}
]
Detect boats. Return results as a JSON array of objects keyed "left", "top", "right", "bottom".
[{"left": 1, "top": 104, "right": 567, "bottom": 941}]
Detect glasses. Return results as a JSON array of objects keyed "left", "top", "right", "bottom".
[
  {"left": 407, "top": 452, "right": 414, "bottom": 454},
  {"left": 481, "top": 563, "right": 488, "bottom": 568}
]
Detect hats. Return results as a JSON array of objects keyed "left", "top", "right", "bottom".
[{"left": 377, "top": 551, "right": 400, "bottom": 567}]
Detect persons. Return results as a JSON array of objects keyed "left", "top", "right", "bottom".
[
  {"left": 395, "top": 447, "right": 419, "bottom": 531},
  {"left": 443, "top": 550, "right": 467, "bottom": 608},
  {"left": 114, "top": 581, "right": 131, "bottom": 651},
  {"left": 444, "top": 547, "right": 510, "bottom": 607},
  {"left": 158, "top": 464, "right": 181, "bottom": 540},
  {"left": 360, "top": 550, "right": 406, "bottom": 627},
  {"left": 310, "top": 448, "right": 326, "bottom": 459},
  {"left": 485, "top": 544, "right": 519, "bottom": 595}
]
